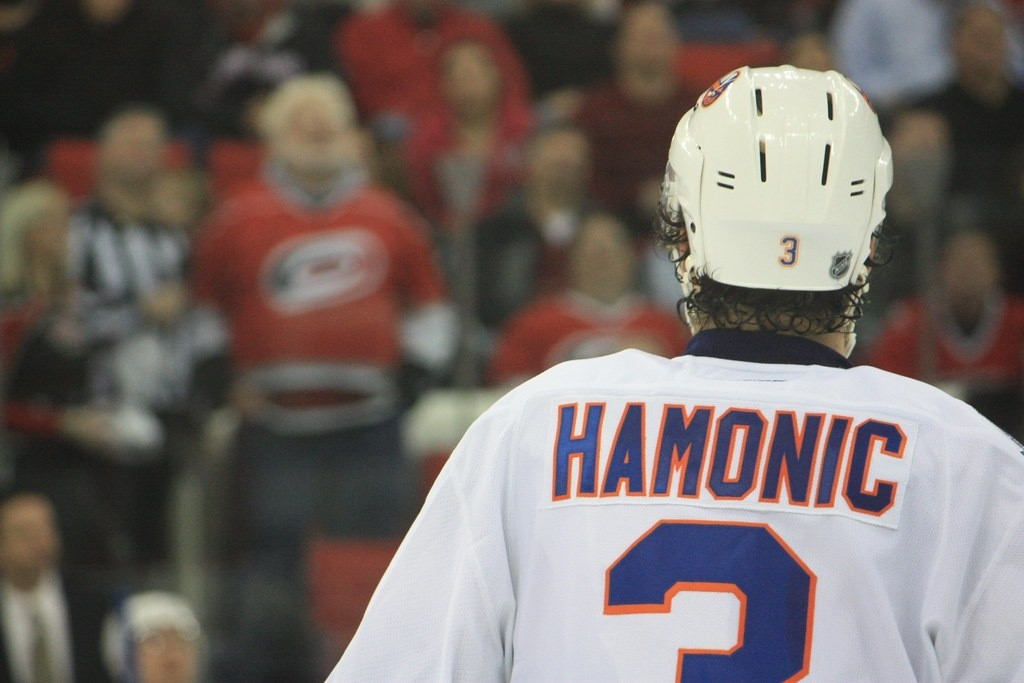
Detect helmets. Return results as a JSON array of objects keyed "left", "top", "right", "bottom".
[
  {"left": 103, "top": 591, "right": 197, "bottom": 680},
  {"left": 661, "top": 66, "right": 894, "bottom": 291}
]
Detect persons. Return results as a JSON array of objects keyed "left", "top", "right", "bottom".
[
  {"left": 489, "top": 217, "right": 692, "bottom": 387},
  {"left": 193, "top": 75, "right": 460, "bottom": 682},
  {"left": 0, "top": 112, "right": 202, "bottom": 683},
  {"left": 0, "top": 0, "right": 1024, "bottom": 332},
  {"left": 0, "top": 492, "right": 103, "bottom": 683},
  {"left": 322, "top": 63, "right": 1024, "bottom": 683},
  {"left": 876, "top": 232, "right": 1024, "bottom": 444},
  {"left": 100, "top": 591, "right": 203, "bottom": 683}
]
